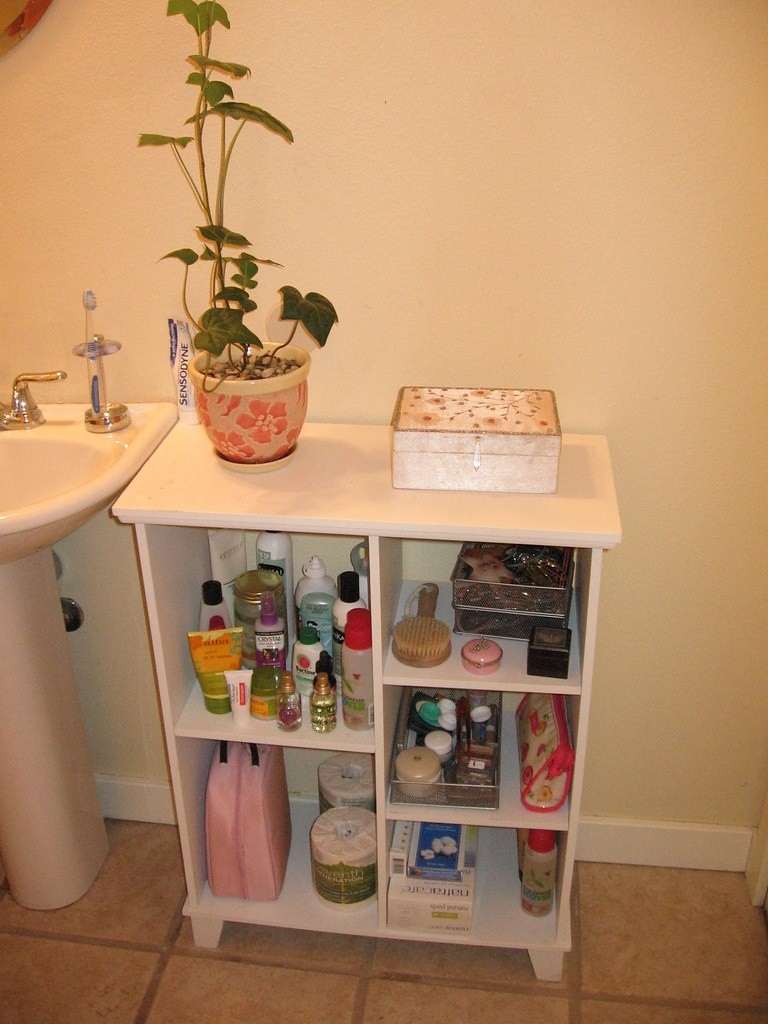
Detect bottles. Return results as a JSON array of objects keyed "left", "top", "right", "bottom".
[
  {"left": 293, "top": 627, "right": 324, "bottom": 697},
  {"left": 300, "top": 592, "right": 336, "bottom": 658},
  {"left": 233, "top": 570, "right": 289, "bottom": 670},
  {"left": 351, "top": 541, "right": 371, "bottom": 611},
  {"left": 309, "top": 651, "right": 338, "bottom": 733},
  {"left": 332, "top": 571, "right": 367, "bottom": 696},
  {"left": 276, "top": 672, "right": 302, "bottom": 733},
  {"left": 254, "top": 591, "right": 286, "bottom": 672},
  {"left": 341, "top": 608, "right": 374, "bottom": 732},
  {"left": 294, "top": 555, "right": 337, "bottom": 634},
  {"left": 517, "top": 828, "right": 558, "bottom": 917},
  {"left": 256, "top": 530, "right": 293, "bottom": 641},
  {"left": 198, "top": 579, "right": 234, "bottom": 632},
  {"left": 250, "top": 648, "right": 284, "bottom": 720}
]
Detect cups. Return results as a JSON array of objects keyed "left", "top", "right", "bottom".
[{"left": 71, "top": 340, "right": 131, "bottom": 433}]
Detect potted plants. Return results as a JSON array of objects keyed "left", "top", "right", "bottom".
[{"left": 137, "top": 0, "right": 340, "bottom": 461}]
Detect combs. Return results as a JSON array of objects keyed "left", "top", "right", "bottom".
[{"left": 392, "top": 582, "right": 453, "bottom": 667}]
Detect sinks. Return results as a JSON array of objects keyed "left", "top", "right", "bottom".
[{"left": 1, "top": 400, "right": 177, "bottom": 570}]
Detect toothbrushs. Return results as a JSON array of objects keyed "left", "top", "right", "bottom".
[{"left": 82, "top": 288, "right": 103, "bottom": 419}]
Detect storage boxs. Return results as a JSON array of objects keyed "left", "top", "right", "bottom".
[
  {"left": 450, "top": 543, "right": 575, "bottom": 642},
  {"left": 527, "top": 626, "right": 572, "bottom": 679},
  {"left": 387, "top": 820, "right": 478, "bottom": 934},
  {"left": 389, "top": 687, "right": 503, "bottom": 810},
  {"left": 391, "top": 386, "right": 563, "bottom": 496}
]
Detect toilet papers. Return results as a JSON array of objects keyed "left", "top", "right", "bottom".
[
  {"left": 317, "top": 752, "right": 377, "bottom": 813},
  {"left": 309, "top": 804, "right": 377, "bottom": 910}
]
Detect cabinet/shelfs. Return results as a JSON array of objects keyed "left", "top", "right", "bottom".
[{"left": 110, "top": 422, "right": 622, "bottom": 983}]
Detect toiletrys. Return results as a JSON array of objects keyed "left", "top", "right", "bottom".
[
  {"left": 186, "top": 530, "right": 373, "bottom": 735},
  {"left": 167, "top": 313, "right": 201, "bottom": 429}
]
